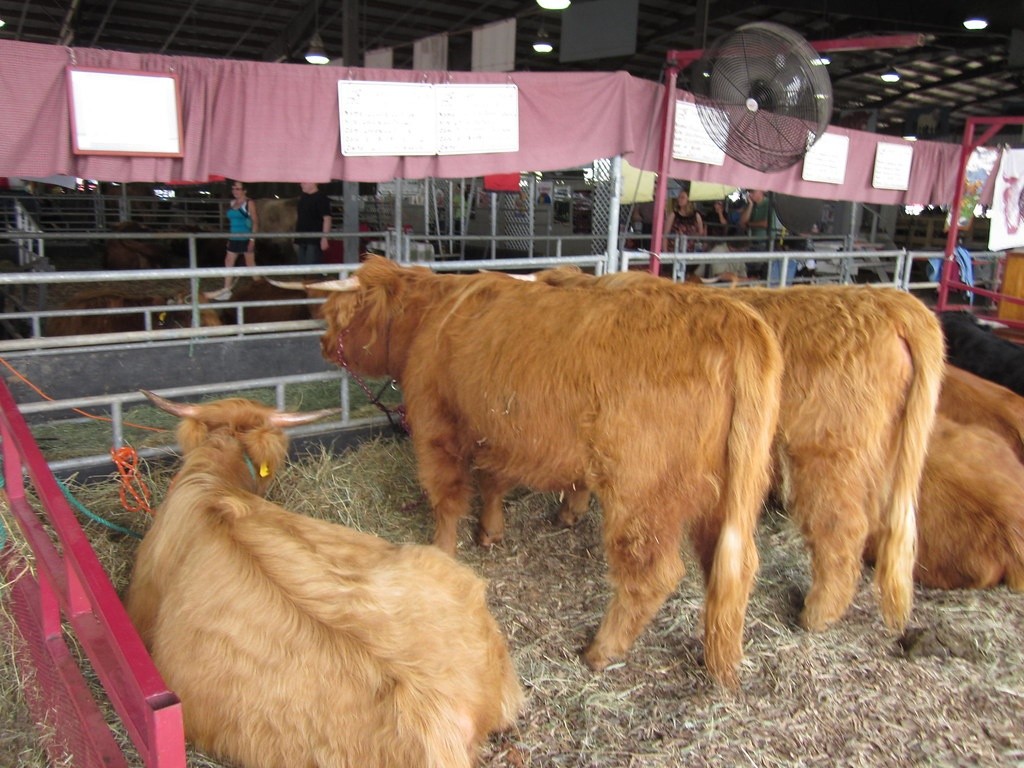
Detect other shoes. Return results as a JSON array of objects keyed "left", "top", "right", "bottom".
[{"left": 214, "top": 291, "right": 231, "bottom": 301}]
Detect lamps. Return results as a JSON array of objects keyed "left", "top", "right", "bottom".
[
  {"left": 533, "top": 7, "right": 553, "bottom": 53},
  {"left": 880, "top": 61, "right": 901, "bottom": 82},
  {"left": 809, "top": 52, "right": 831, "bottom": 65},
  {"left": 304, "top": 0, "right": 330, "bottom": 64},
  {"left": 964, "top": 6, "right": 988, "bottom": 29}
]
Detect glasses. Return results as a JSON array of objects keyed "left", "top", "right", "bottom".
[{"left": 232, "top": 188, "right": 244, "bottom": 191}]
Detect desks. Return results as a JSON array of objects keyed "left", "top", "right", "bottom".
[{"left": 370, "top": 239, "right": 434, "bottom": 274}]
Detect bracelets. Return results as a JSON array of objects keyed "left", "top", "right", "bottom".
[{"left": 250, "top": 238, "right": 256, "bottom": 241}]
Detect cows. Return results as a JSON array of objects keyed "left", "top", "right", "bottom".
[
  {"left": 47, "top": 198, "right": 300, "bottom": 272},
  {"left": 479, "top": 264, "right": 946, "bottom": 637},
  {"left": 863, "top": 310, "right": 1024, "bottom": 594},
  {"left": 0, "top": 257, "right": 317, "bottom": 339},
  {"left": 122, "top": 388, "right": 526, "bottom": 768},
  {"left": 305, "top": 252, "right": 782, "bottom": 677}
]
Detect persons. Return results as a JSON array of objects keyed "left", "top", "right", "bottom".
[
  {"left": 632, "top": 177, "right": 821, "bottom": 285},
  {"left": 293, "top": 181, "right": 332, "bottom": 313},
  {"left": 213, "top": 178, "right": 260, "bottom": 299},
  {"left": 452, "top": 183, "right": 468, "bottom": 243}
]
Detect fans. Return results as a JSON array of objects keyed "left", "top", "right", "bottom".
[{"left": 694, "top": 21, "right": 833, "bottom": 173}]
